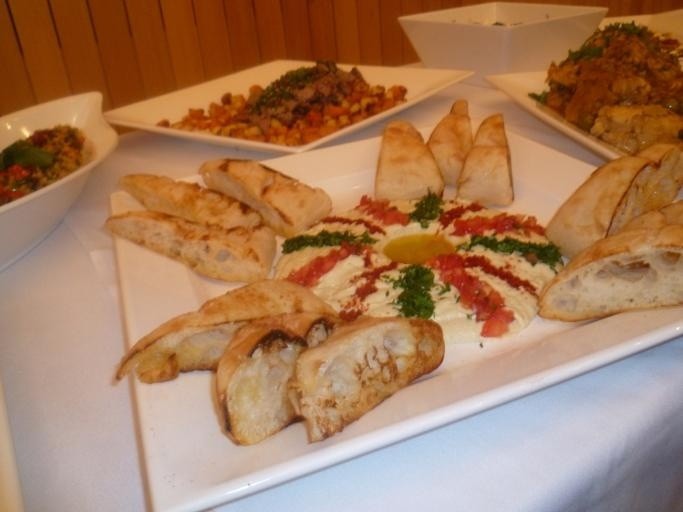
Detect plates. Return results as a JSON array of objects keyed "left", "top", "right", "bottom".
[
  {"left": 598, "top": 8, "right": 683, "bottom": 75},
  {"left": 106, "top": 59, "right": 473, "bottom": 156},
  {"left": 107, "top": 105, "right": 683, "bottom": 512},
  {"left": 482, "top": 69, "right": 630, "bottom": 166}
]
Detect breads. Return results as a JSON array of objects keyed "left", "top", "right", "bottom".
[{"left": 104, "top": 98, "right": 683, "bottom": 446}]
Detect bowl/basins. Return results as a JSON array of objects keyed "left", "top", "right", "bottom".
[
  {"left": 397, "top": 0, "right": 610, "bottom": 75},
  {"left": 0, "top": 91, "right": 121, "bottom": 277}
]
